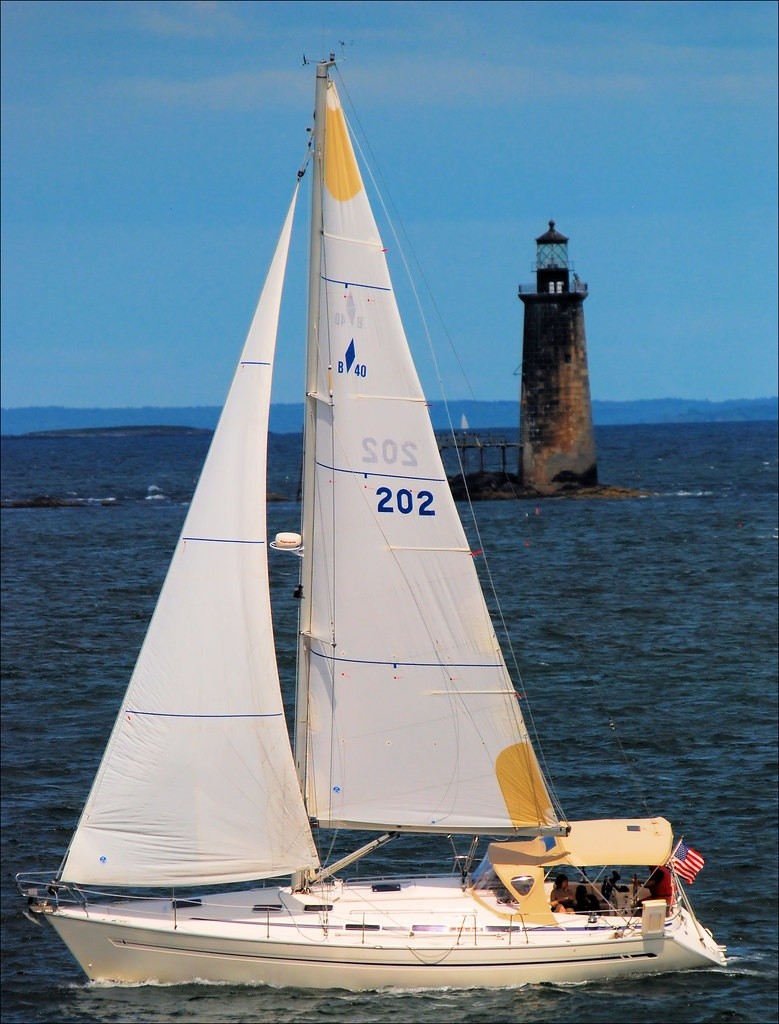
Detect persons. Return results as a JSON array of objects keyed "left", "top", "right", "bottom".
[
  {"left": 572, "top": 885, "right": 601, "bottom": 915},
  {"left": 609, "top": 870, "right": 621, "bottom": 887},
  {"left": 550, "top": 874, "right": 577, "bottom": 914},
  {"left": 602, "top": 875, "right": 612, "bottom": 903},
  {"left": 634, "top": 865, "right": 671, "bottom": 917}
]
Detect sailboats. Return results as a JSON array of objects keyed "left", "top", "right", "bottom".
[{"left": 14, "top": 48, "right": 728, "bottom": 993}]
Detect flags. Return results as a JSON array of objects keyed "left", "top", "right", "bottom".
[{"left": 665, "top": 839, "right": 706, "bottom": 885}]
